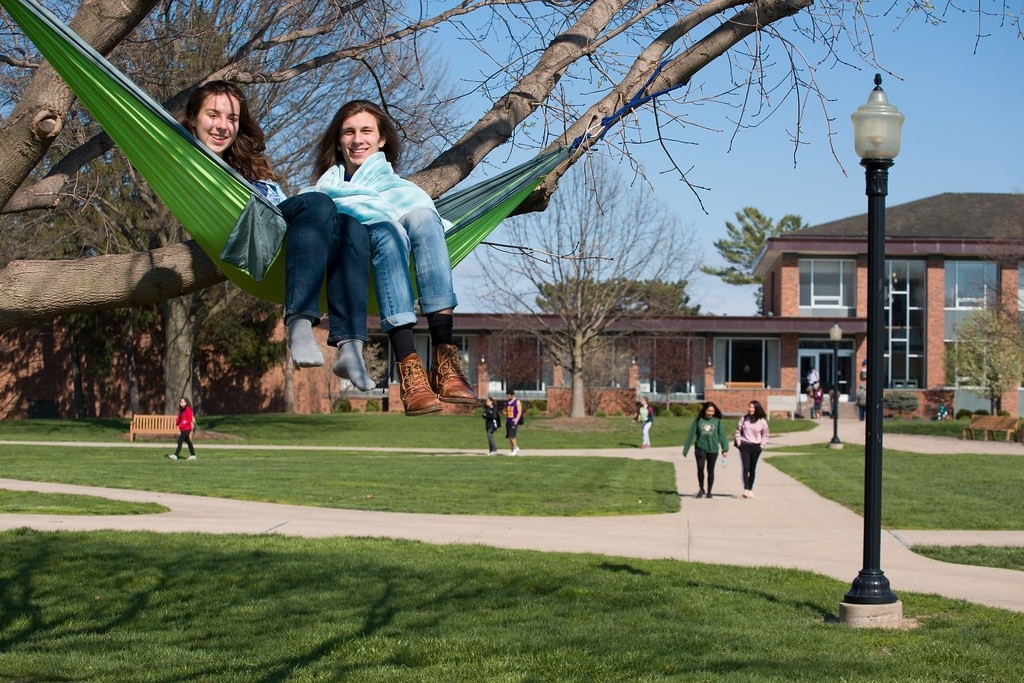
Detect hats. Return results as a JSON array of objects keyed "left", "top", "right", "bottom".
[{"left": 859, "top": 384, "right": 864, "bottom": 389}]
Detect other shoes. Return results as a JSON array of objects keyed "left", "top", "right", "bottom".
[
  {"left": 743, "top": 490, "right": 753, "bottom": 498},
  {"left": 169, "top": 454, "right": 177, "bottom": 460},
  {"left": 696, "top": 489, "right": 704, "bottom": 498},
  {"left": 707, "top": 491, "right": 712, "bottom": 497},
  {"left": 508, "top": 447, "right": 519, "bottom": 456},
  {"left": 186, "top": 455, "right": 196, "bottom": 460},
  {"left": 640, "top": 444, "right": 648, "bottom": 448},
  {"left": 487, "top": 451, "right": 495, "bottom": 455}
]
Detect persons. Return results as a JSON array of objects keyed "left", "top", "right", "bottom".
[
  {"left": 482, "top": 397, "right": 499, "bottom": 456},
  {"left": 682, "top": 402, "right": 729, "bottom": 498},
  {"left": 168, "top": 397, "right": 196, "bottom": 460},
  {"left": 170, "top": 79, "right": 376, "bottom": 392},
  {"left": 828, "top": 385, "right": 841, "bottom": 418},
  {"left": 807, "top": 381, "right": 823, "bottom": 419},
  {"left": 311, "top": 100, "right": 476, "bottom": 416},
  {"left": 632, "top": 397, "right": 654, "bottom": 449},
  {"left": 502, "top": 389, "right": 522, "bottom": 456},
  {"left": 735, "top": 400, "right": 769, "bottom": 498},
  {"left": 806, "top": 367, "right": 820, "bottom": 387},
  {"left": 857, "top": 384, "right": 866, "bottom": 421}
]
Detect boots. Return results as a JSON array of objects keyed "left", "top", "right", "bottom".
[
  {"left": 426, "top": 343, "right": 478, "bottom": 403},
  {"left": 396, "top": 351, "right": 443, "bottom": 415}
]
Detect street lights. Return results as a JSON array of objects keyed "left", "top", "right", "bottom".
[
  {"left": 829, "top": 324, "right": 844, "bottom": 450},
  {"left": 838, "top": 72, "right": 905, "bottom": 629}
]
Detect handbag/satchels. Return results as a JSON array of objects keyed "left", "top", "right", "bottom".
[
  {"left": 517, "top": 415, "right": 523, "bottom": 425},
  {"left": 734, "top": 416, "right": 746, "bottom": 448}
]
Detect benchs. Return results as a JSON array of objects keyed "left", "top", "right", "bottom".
[
  {"left": 129, "top": 414, "right": 195, "bottom": 442},
  {"left": 963, "top": 414, "right": 1019, "bottom": 443}
]
